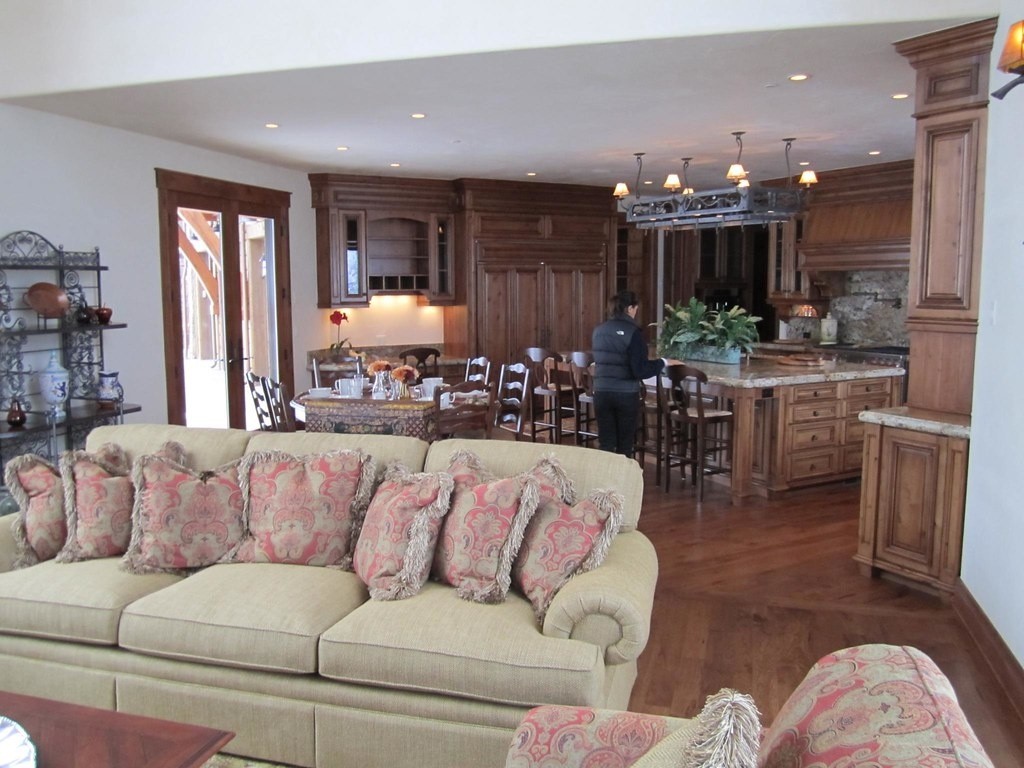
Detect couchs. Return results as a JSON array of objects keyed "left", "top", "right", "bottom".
[
  {"left": 506, "top": 645, "right": 997, "bottom": 767},
  {"left": 1, "top": 424, "right": 658, "bottom": 766}
]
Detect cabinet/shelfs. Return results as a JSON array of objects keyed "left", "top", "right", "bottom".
[
  {"left": 308, "top": 172, "right": 459, "bottom": 305},
  {"left": 852, "top": 408, "right": 972, "bottom": 605},
  {"left": 732, "top": 367, "right": 907, "bottom": 498},
  {"left": 454, "top": 177, "right": 659, "bottom": 407}
]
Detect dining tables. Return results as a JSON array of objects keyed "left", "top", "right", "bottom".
[{"left": 293, "top": 381, "right": 453, "bottom": 441}]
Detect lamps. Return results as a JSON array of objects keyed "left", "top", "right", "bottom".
[
  {"left": 991, "top": 20, "right": 1024, "bottom": 98},
  {"left": 612, "top": 131, "right": 818, "bottom": 199}
]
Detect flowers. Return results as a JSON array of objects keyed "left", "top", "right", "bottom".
[
  {"left": 329, "top": 311, "right": 352, "bottom": 357},
  {"left": 367, "top": 360, "right": 419, "bottom": 382}
]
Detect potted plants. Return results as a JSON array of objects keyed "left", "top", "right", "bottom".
[{"left": 648, "top": 297, "right": 763, "bottom": 364}]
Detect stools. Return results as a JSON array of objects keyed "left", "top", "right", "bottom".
[{"left": 522, "top": 344, "right": 733, "bottom": 503}]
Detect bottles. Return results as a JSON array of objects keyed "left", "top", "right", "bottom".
[
  {"left": 38, "top": 351, "right": 70, "bottom": 418},
  {"left": 98, "top": 370, "right": 124, "bottom": 404},
  {"left": 6, "top": 400, "right": 27, "bottom": 427}
]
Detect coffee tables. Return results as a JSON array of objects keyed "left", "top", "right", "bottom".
[{"left": 0, "top": 691, "right": 236, "bottom": 768}]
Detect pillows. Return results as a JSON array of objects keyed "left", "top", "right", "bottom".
[
  {"left": 629, "top": 686, "right": 763, "bottom": 767},
  {"left": 2, "top": 440, "right": 623, "bottom": 628}
]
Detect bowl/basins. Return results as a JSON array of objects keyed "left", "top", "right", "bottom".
[{"left": 308, "top": 387, "right": 332, "bottom": 398}]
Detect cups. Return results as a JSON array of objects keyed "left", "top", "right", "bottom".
[
  {"left": 422, "top": 377, "right": 443, "bottom": 402},
  {"left": 440, "top": 383, "right": 456, "bottom": 408},
  {"left": 353, "top": 373, "right": 363, "bottom": 399},
  {"left": 388, "top": 371, "right": 401, "bottom": 401},
  {"left": 335, "top": 378, "right": 353, "bottom": 398},
  {"left": 821, "top": 312, "right": 837, "bottom": 342}
]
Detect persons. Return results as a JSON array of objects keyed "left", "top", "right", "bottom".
[{"left": 592, "top": 292, "right": 685, "bottom": 458}]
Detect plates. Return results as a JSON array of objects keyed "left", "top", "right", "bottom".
[{"left": 27, "top": 282, "right": 70, "bottom": 317}]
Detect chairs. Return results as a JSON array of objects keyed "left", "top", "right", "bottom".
[
  {"left": 246, "top": 372, "right": 296, "bottom": 432},
  {"left": 400, "top": 347, "right": 533, "bottom": 437}
]
